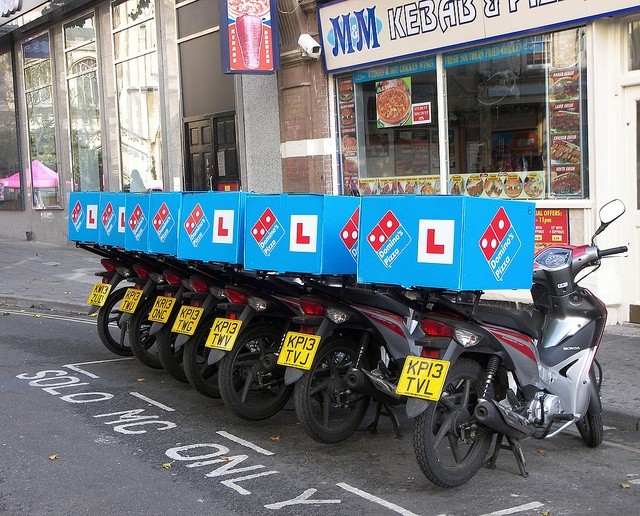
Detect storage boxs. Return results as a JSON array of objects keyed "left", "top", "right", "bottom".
[
  {"left": 68, "top": 192, "right": 97, "bottom": 244},
  {"left": 98, "top": 191, "right": 126, "bottom": 248},
  {"left": 177, "top": 192, "right": 243, "bottom": 262},
  {"left": 244, "top": 191, "right": 360, "bottom": 275},
  {"left": 357, "top": 194, "right": 536, "bottom": 291},
  {"left": 125, "top": 193, "right": 148, "bottom": 253},
  {"left": 149, "top": 194, "right": 179, "bottom": 255}
]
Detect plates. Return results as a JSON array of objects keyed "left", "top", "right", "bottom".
[
  {"left": 551, "top": 172, "right": 582, "bottom": 196},
  {"left": 551, "top": 134, "right": 580, "bottom": 165}
]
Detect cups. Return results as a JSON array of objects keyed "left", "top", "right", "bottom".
[{"left": 235, "top": 14, "right": 264, "bottom": 69}]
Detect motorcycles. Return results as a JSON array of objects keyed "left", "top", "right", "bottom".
[
  {"left": 176, "top": 261, "right": 219, "bottom": 402},
  {"left": 277, "top": 276, "right": 423, "bottom": 446},
  {"left": 358, "top": 198, "right": 628, "bottom": 489},
  {"left": 118, "top": 251, "right": 165, "bottom": 372},
  {"left": 204, "top": 269, "right": 293, "bottom": 423},
  {"left": 147, "top": 253, "right": 191, "bottom": 387},
  {"left": 70, "top": 239, "right": 157, "bottom": 358}
]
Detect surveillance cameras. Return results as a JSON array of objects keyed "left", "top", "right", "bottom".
[{"left": 297, "top": 32, "right": 322, "bottom": 60}]
